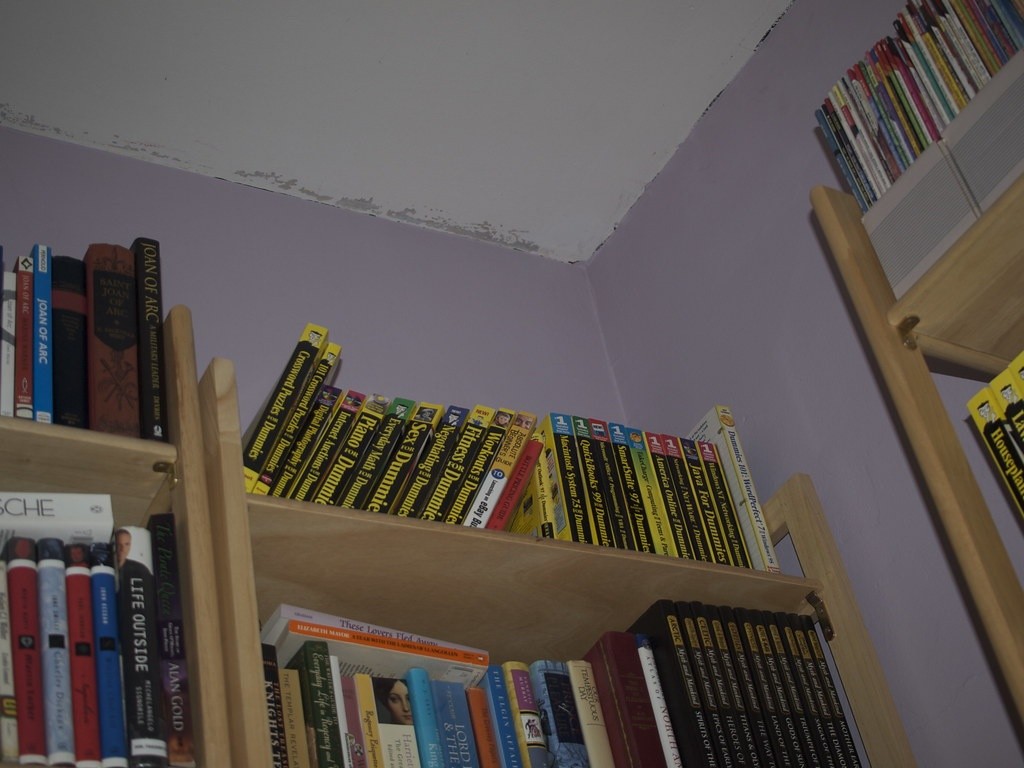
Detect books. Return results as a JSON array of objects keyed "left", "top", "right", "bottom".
[
  {"left": 243, "top": 322, "right": 781, "bottom": 573},
  {"left": 815, "top": 0, "right": 1024, "bottom": 214},
  {"left": 1, "top": 491, "right": 196, "bottom": 768},
  {"left": 260, "top": 599, "right": 862, "bottom": 768},
  {"left": 967, "top": 352, "right": 1024, "bottom": 519},
  {"left": 0, "top": 237, "right": 169, "bottom": 441}
]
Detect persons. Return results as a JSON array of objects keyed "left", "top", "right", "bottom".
[
  {"left": 515, "top": 414, "right": 533, "bottom": 429},
  {"left": 372, "top": 677, "right": 413, "bottom": 725},
  {"left": 494, "top": 414, "right": 510, "bottom": 428},
  {"left": 414, "top": 409, "right": 434, "bottom": 422},
  {"left": 115, "top": 529, "right": 163, "bottom": 737}
]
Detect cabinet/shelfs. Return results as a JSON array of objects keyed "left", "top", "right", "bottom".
[
  {"left": 809, "top": 176, "right": 1024, "bottom": 724},
  {"left": 198, "top": 358, "right": 918, "bottom": 768},
  {"left": 0, "top": 303, "right": 234, "bottom": 768}
]
